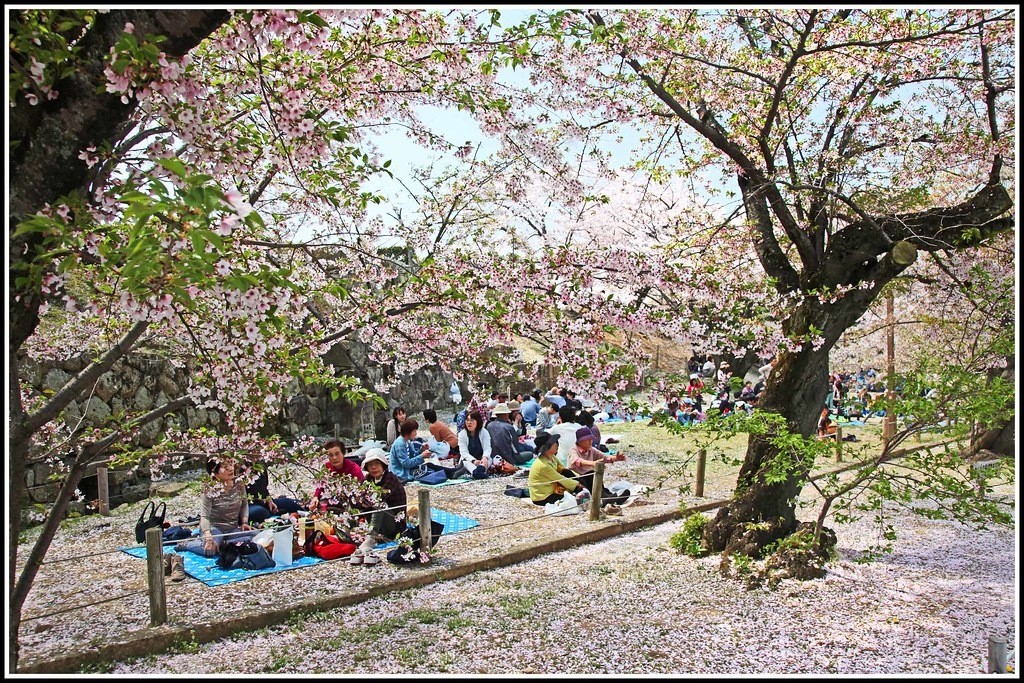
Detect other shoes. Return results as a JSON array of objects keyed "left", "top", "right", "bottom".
[
  {"left": 602, "top": 503, "right": 622, "bottom": 515},
  {"left": 179, "top": 516, "right": 200, "bottom": 526},
  {"left": 605, "top": 438, "right": 619, "bottom": 443},
  {"left": 350, "top": 549, "right": 364, "bottom": 564},
  {"left": 364, "top": 548, "right": 382, "bottom": 564}
]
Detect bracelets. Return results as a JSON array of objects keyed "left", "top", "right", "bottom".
[
  {"left": 241, "top": 522, "right": 248, "bottom": 524},
  {"left": 578, "top": 484, "right": 581, "bottom": 489}
]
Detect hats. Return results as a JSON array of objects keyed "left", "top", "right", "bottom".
[
  {"left": 534, "top": 432, "right": 560, "bottom": 458},
  {"left": 552, "top": 403, "right": 559, "bottom": 412},
  {"left": 477, "top": 382, "right": 489, "bottom": 390},
  {"left": 360, "top": 448, "right": 389, "bottom": 471},
  {"left": 493, "top": 403, "right": 511, "bottom": 414},
  {"left": 575, "top": 427, "right": 597, "bottom": 443},
  {"left": 507, "top": 401, "right": 521, "bottom": 410}
]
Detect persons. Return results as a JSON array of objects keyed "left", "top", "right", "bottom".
[{"left": 174, "top": 357, "right": 935, "bottom": 559}]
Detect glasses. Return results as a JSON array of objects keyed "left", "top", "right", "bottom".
[{"left": 465, "top": 419, "right": 475, "bottom": 422}]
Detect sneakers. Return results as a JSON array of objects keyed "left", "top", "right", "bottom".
[
  {"left": 171, "top": 555, "right": 185, "bottom": 580},
  {"left": 163, "top": 552, "right": 176, "bottom": 574}
]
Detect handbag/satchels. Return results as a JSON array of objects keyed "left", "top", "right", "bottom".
[
  {"left": 135, "top": 501, "right": 166, "bottom": 544},
  {"left": 229, "top": 544, "right": 276, "bottom": 570},
  {"left": 428, "top": 435, "right": 450, "bottom": 458},
  {"left": 386, "top": 521, "right": 445, "bottom": 564},
  {"left": 450, "top": 382, "right": 460, "bottom": 393},
  {"left": 303, "top": 530, "right": 357, "bottom": 561},
  {"left": 413, "top": 465, "right": 447, "bottom": 485},
  {"left": 494, "top": 461, "right": 519, "bottom": 473}
]
